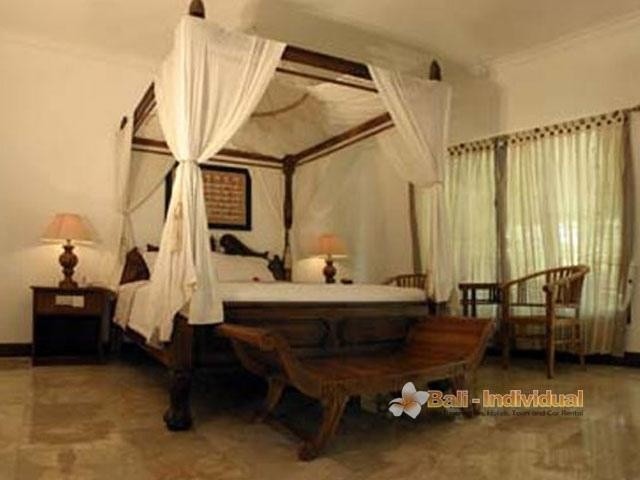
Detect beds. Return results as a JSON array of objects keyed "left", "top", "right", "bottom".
[{"left": 112, "top": 0, "right": 455, "bottom": 429}]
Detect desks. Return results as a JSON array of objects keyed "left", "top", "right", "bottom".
[{"left": 459, "top": 283, "right": 499, "bottom": 317}]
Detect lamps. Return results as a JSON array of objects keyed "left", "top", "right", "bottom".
[
  {"left": 311, "top": 235, "right": 349, "bottom": 283},
  {"left": 39, "top": 214, "right": 95, "bottom": 288}
]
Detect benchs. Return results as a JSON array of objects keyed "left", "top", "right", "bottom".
[{"left": 214, "top": 313, "right": 498, "bottom": 462}]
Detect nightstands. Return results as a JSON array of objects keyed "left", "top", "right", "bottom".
[{"left": 29, "top": 285, "right": 116, "bottom": 367}]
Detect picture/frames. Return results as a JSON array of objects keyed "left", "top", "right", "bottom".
[{"left": 165, "top": 164, "right": 251, "bottom": 231}]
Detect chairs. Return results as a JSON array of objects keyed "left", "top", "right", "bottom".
[
  {"left": 498, "top": 264, "right": 590, "bottom": 379},
  {"left": 382, "top": 273, "right": 434, "bottom": 316}
]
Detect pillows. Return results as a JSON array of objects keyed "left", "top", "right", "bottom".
[
  {"left": 211, "top": 251, "right": 276, "bottom": 282},
  {"left": 121, "top": 247, "right": 149, "bottom": 285},
  {"left": 142, "top": 250, "right": 159, "bottom": 277}
]
